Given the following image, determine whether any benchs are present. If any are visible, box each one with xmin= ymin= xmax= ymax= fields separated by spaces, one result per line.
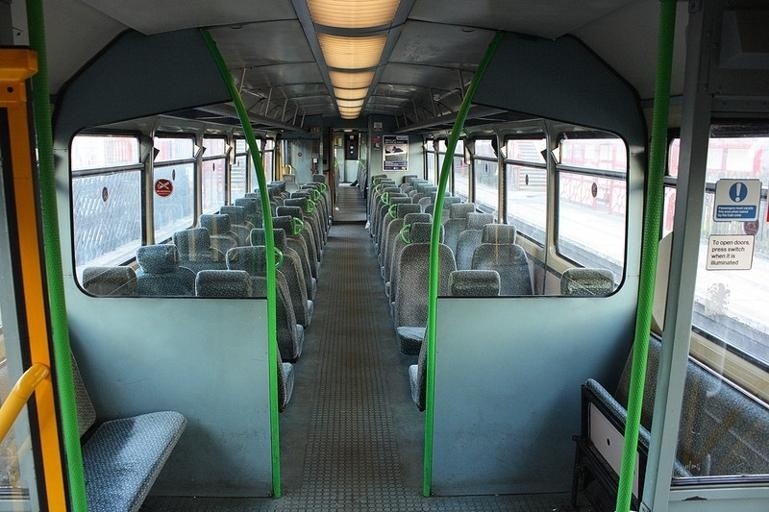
xmin=66 ymin=336 xmax=194 ymax=512
xmin=571 ymin=323 xmax=768 ymax=512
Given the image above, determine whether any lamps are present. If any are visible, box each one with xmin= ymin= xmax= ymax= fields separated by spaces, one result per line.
xmin=291 ymin=1 xmax=414 ymax=127
